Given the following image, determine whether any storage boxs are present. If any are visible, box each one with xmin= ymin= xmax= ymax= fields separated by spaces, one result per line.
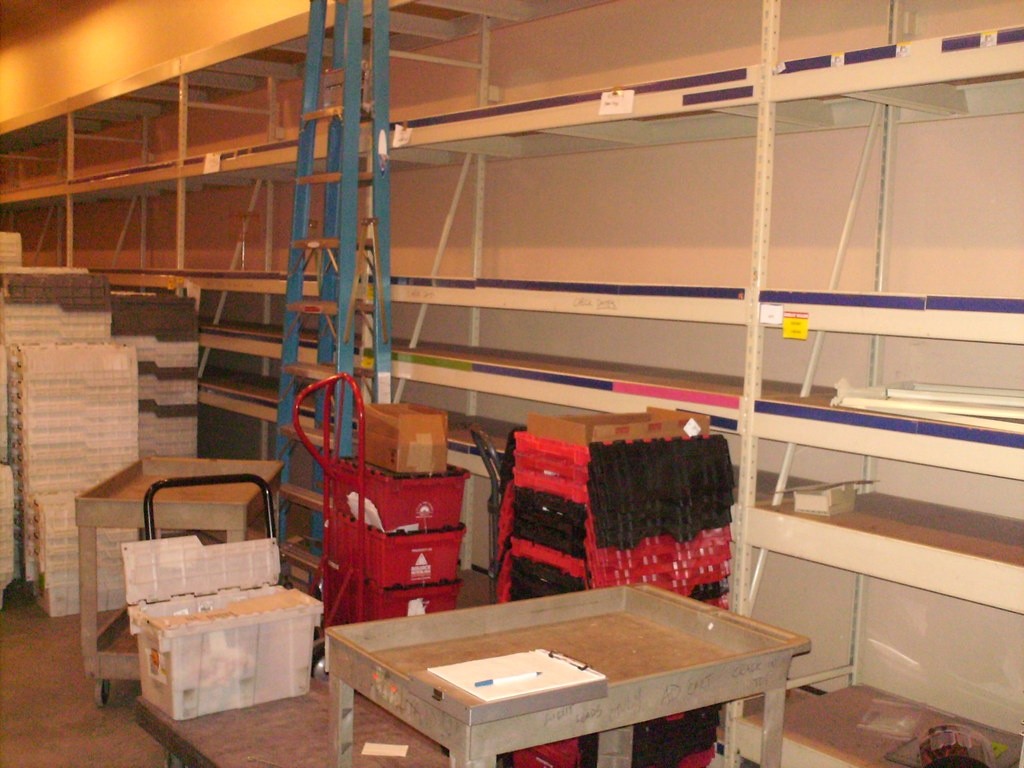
xmin=0 ymin=230 xmax=735 ymax=768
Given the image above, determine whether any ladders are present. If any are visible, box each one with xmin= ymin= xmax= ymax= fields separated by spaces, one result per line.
xmin=272 ymin=0 xmax=391 ymax=682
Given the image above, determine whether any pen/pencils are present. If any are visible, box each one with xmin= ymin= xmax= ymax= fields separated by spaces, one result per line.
xmin=475 ymin=671 xmax=542 ymax=687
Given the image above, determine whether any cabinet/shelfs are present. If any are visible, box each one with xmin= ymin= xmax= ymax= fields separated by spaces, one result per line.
xmin=1 ymin=0 xmax=1023 ymax=767
